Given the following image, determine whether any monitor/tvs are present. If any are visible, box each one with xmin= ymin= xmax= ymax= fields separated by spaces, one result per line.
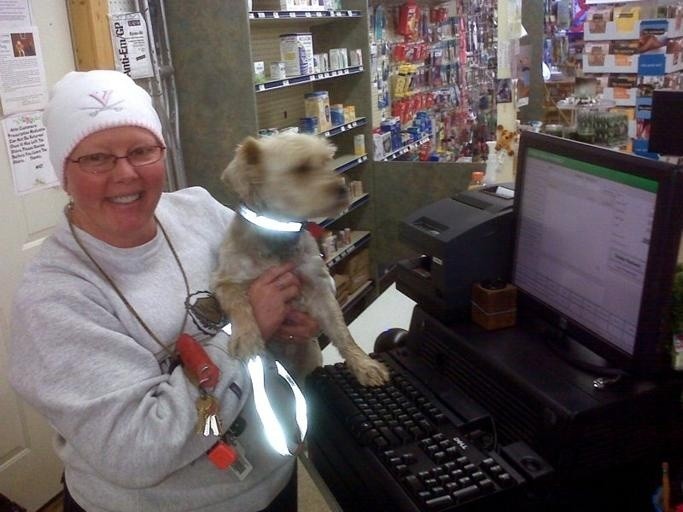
xmin=502 ymin=130 xmax=683 ymax=382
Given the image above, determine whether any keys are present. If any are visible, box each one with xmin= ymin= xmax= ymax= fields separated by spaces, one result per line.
xmin=194 ymin=393 xmax=224 ymax=436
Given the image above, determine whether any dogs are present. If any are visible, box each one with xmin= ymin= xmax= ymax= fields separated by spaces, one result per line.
xmin=209 ymin=127 xmax=390 ymax=458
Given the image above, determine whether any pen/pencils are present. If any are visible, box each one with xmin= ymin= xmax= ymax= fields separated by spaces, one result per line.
xmin=661 ymin=462 xmax=671 ymax=512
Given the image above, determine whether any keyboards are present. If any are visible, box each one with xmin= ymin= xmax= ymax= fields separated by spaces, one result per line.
xmin=309 ymin=351 xmax=525 ymax=512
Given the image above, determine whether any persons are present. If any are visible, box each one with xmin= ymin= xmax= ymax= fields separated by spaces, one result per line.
xmin=6 ymin=67 xmax=325 ymax=512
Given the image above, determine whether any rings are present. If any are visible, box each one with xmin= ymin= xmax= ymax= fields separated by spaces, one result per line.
xmin=288 ymin=334 xmax=296 ymax=345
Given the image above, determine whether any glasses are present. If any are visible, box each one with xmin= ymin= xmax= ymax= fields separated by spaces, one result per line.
xmin=67 ymin=145 xmax=166 ymax=175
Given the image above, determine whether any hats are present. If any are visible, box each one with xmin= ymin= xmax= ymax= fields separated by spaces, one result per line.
xmin=41 ymin=70 xmax=166 ymax=190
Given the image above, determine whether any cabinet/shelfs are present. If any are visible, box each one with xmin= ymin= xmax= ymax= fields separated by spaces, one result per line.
xmin=159 ymin=2 xmax=380 ymax=351
xmin=381 ymin=112 xmax=434 ymax=167
xmin=521 ymin=3 xmax=569 ymax=139
